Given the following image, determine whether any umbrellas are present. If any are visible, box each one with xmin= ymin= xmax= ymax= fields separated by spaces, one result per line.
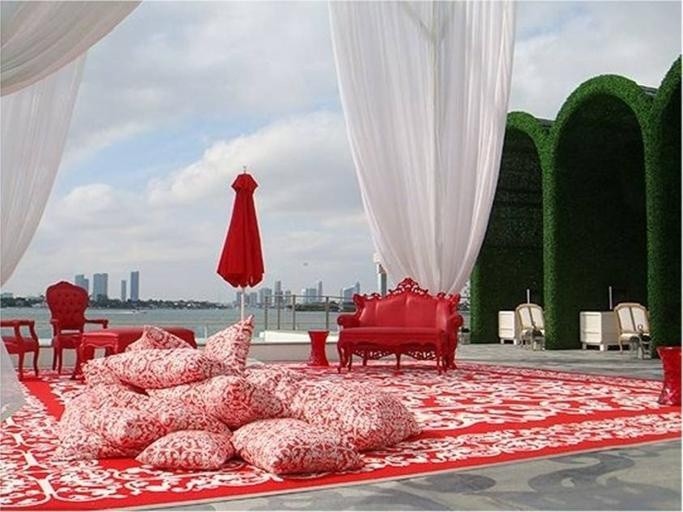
xmin=212 ymin=163 xmax=265 ymax=325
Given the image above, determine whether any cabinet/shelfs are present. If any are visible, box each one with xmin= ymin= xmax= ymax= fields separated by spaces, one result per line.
xmin=498 ymin=310 xmax=522 ymax=345
xmin=579 ymin=309 xmax=627 ymax=351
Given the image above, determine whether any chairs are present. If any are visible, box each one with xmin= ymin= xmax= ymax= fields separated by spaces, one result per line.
xmin=517 ymin=303 xmax=546 ymax=348
xmin=615 ymin=304 xmax=653 ymax=356
xmin=0 ymin=279 xmax=109 ymax=379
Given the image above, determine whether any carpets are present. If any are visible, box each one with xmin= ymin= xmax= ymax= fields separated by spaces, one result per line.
xmin=0 ymin=355 xmax=683 ymax=511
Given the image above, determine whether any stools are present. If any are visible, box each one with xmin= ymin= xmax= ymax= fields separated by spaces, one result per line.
xmin=657 ymin=346 xmax=682 ymax=406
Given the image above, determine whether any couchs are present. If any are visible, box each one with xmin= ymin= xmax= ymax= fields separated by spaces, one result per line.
xmin=334 ymin=276 xmax=463 ymax=376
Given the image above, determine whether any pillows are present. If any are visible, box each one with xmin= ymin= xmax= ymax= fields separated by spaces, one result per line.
xmin=52 ymin=313 xmax=295 ymax=458
xmin=136 ymin=430 xmax=232 ymax=472
xmin=294 ymin=382 xmax=421 ymax=446
xmin=232 ymin=421 xmax=363 ymax=472
